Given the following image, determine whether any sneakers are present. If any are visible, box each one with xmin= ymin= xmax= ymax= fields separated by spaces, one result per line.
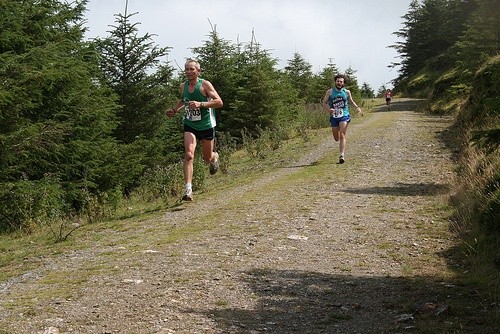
xmin=209 ymin=152 xmax=219 ymax=175
xmin=182 ymin=188 xmax=193 ymax=202
xmin=338 ymin=156 xmax=345 ymax=164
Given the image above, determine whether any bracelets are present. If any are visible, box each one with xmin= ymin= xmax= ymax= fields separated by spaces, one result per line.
xmin=172 ymin=107 xmax=177 ymax=114
xmin=355 ymin=106 xmax=358 ymax=109
xmin=200 ymin=102 xmax=204 ymax=107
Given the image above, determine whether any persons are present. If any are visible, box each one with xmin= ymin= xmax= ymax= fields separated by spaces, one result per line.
xmin=323 ymin=74 xmax=361 ymax=163
xmin=384 ymin=89 xmax=392 ymax=111
xmin=165 ymin=59 xmax=223 ymax=201
